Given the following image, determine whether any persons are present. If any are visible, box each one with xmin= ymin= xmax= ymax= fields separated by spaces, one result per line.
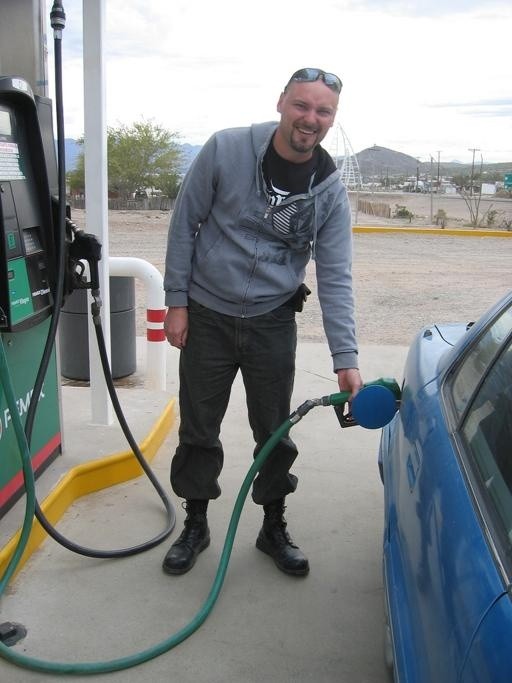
xmin=160 ymin=67 xmax=363 ymax=576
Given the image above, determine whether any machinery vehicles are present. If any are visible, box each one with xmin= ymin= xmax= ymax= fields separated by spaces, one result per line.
xmin=414 ymin=180 xmax=440 ymax=193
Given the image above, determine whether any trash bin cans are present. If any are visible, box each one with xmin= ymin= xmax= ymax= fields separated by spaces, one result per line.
xmin=58 ymin=276 xmax=136 ymax=381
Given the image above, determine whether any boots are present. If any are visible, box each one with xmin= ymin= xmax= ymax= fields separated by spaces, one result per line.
xmin=163 ymin=499 xmax=210 ymax=575
xmin=256 ymin=497 xmax=309 ymax=575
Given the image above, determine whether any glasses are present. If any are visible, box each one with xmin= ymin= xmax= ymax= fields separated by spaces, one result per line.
xmin=283 ymin=67 xmax=343 ymax=95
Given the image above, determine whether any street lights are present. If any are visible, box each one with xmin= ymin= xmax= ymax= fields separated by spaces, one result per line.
xmin=468 ymin=149 xmax=480 ymax=196
xmin=416 ymin=156 xmax=421 ymax=193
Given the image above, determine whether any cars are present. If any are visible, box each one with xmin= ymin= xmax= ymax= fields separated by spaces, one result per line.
xmin=374 ymin=288 xmax=512 ymax=683
